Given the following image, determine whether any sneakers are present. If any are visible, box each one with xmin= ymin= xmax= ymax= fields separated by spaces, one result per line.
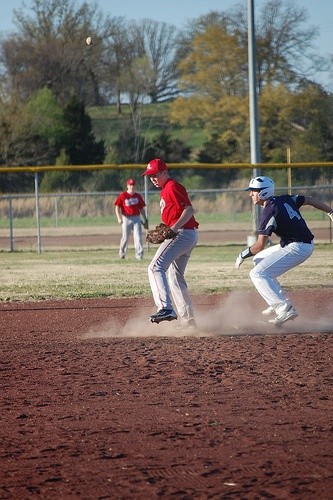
xmin=268 ymin=305 xmax=298 ymax=327
xmin=150 ymin=309 xmax=178 ymax=324
xmin=262 ymin=307 xmax=275 ymax=315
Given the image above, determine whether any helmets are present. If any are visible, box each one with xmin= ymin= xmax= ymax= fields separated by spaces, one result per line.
xmin=245 ymin=176 xmax=275 ymax=200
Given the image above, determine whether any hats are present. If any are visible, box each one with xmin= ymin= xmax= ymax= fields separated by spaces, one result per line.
xmin=141 ymin=159 xmax=168 ymax=176
xmin=127 ymin=179 xmax=136 ymax=185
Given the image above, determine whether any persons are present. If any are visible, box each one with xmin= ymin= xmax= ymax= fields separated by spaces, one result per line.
xmin=234 ymin=177 xmax=333 ymax=324
xmin=114 ymin=180 xmax=148 ymax=262
xmin=141 ymin=159 xmax=199 ymax=333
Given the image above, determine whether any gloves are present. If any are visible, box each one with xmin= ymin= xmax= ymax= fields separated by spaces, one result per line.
xmin=235 ymin=247 xmax=256 ymax=270
xmin=328 ymin=213 xmax=333 ymax=221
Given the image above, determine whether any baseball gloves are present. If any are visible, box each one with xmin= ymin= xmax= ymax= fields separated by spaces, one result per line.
xmin=145 ymin=223 xmax=178 ymax=244
